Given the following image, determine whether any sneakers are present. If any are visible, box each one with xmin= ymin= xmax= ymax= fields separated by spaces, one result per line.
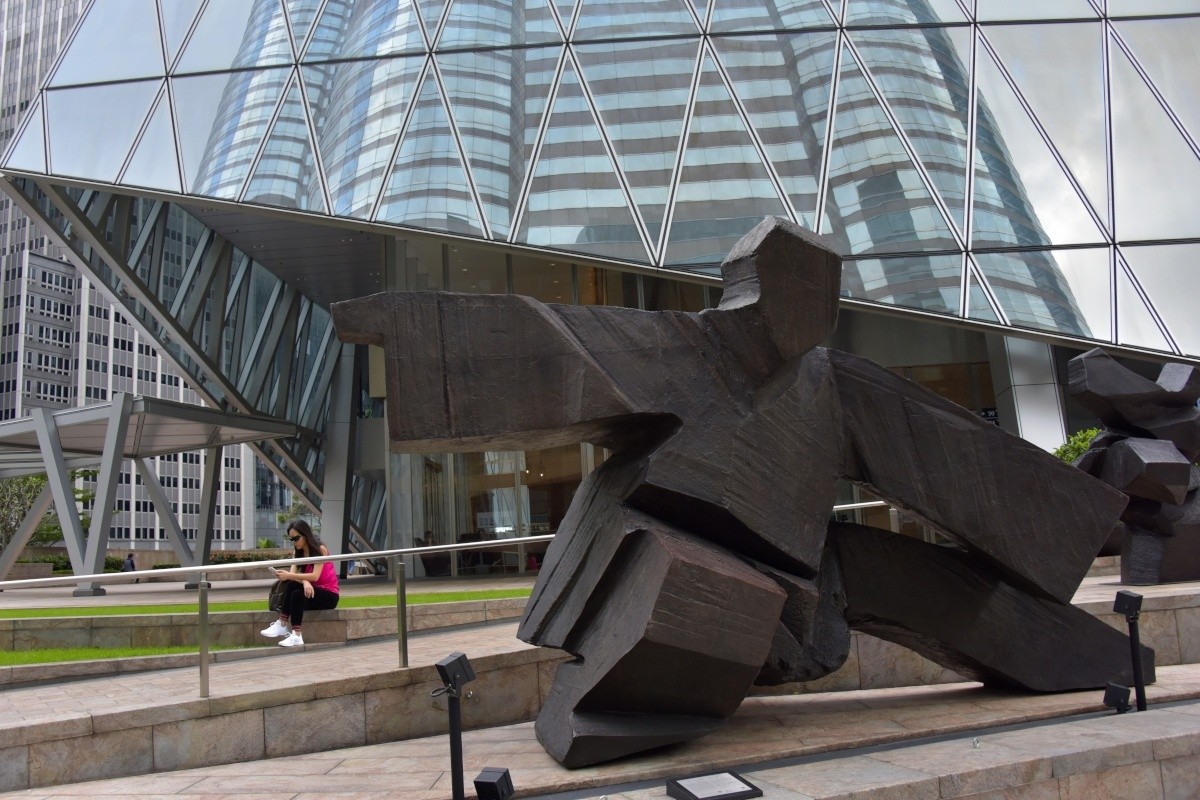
xmin=278 ymin=629 xmax=305 ymax=647
xmin=260 ymin=619 xmax=289 ymax=638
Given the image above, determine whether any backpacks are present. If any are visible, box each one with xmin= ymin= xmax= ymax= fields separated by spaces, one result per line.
xmin=268 ymin=580 xmax=290 ymax=612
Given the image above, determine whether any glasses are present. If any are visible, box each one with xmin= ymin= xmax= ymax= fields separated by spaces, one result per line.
xmin=288 ymin=534 xmax=304 ymax=542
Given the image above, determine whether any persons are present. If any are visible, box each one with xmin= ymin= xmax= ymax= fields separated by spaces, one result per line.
xmin=124 ymin=553 xmax=140 ymax=584
xmin=423 ymin=531 xmax=450 ymax=558
xmin=260 ymin=520 xmax=339 ymax=647
xmin=347 ymin=560 xmax=355 ymax=576
xmin=210 ymin=553 xmax=216 ymax=561
xmin=457 ymin=534 xmax=474 ymax=566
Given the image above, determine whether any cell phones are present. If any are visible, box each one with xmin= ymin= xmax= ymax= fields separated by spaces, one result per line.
xmin=268 ymin=567 xmax=277 ymax=574
